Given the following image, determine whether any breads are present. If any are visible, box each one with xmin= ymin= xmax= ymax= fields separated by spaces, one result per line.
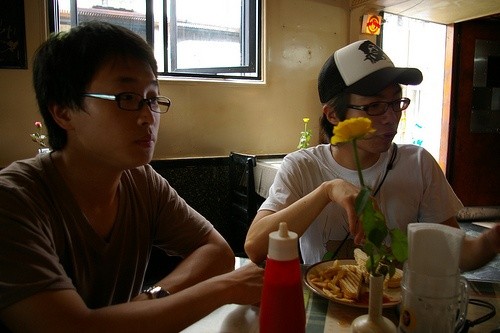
xmin=339 ymin=248 xmax=404 ymax=299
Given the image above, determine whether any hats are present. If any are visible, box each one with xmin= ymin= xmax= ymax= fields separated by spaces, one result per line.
xmin=318 ymin=40 xmax=423 ymax=102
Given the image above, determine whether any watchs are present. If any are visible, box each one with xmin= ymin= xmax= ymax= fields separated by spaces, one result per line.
xmin=143 ymin=286 xmax=170 ymax=298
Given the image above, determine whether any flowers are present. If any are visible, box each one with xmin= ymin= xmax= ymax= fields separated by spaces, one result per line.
xmin=31 ymin=122 xmax=46 ymax=150
xmin=331 ymin=117 xmax=409 ymax=278
xmin=298 ymin=117 xmax=312 ymax=149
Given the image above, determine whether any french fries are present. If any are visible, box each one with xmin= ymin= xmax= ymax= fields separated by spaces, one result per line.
xmin=309 ymin=259 xmax=357 ymax=303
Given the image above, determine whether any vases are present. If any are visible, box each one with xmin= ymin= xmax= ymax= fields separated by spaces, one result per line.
xmin=350 ymin=273 xmax=397 ymax=333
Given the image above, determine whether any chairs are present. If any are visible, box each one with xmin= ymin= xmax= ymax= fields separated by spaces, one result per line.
xmin=227 ymin=152 xmax=256 ymax=252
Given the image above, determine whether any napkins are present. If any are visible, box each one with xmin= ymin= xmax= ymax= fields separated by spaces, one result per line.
xmin=407 ymin=222 xmax=465 ymax=297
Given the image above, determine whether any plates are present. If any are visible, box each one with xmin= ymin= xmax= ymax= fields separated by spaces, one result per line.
xmin=303 ymin=259 xmax=405 ymax=308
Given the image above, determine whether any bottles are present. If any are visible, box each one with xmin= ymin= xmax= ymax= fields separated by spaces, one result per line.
xmin=258 ymin=222 xmax=306 ymax=333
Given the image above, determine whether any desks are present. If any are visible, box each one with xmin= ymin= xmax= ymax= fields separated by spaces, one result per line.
xmin=253 ymin=158 xmax=286 ymax=198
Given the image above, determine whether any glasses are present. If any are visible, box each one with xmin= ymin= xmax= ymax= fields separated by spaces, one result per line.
xmin=80 ymin=92 xmax=171 ymax=115
xmin=345 ymin=96 xmax=410 ymax=116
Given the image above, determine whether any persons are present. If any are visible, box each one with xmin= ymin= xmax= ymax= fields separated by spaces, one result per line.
xmin=0 ymin=22 xmax=264 ymax=332
xmin=243 ymin=40 xmax=464 ymax=263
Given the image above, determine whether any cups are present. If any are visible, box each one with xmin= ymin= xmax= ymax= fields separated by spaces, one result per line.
xmin=397 ymin=274 xmax=469 ymax=333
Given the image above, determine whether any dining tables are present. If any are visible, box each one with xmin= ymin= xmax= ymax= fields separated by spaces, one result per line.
xmin=181 ymin=257 xmax=500 ymax=333
